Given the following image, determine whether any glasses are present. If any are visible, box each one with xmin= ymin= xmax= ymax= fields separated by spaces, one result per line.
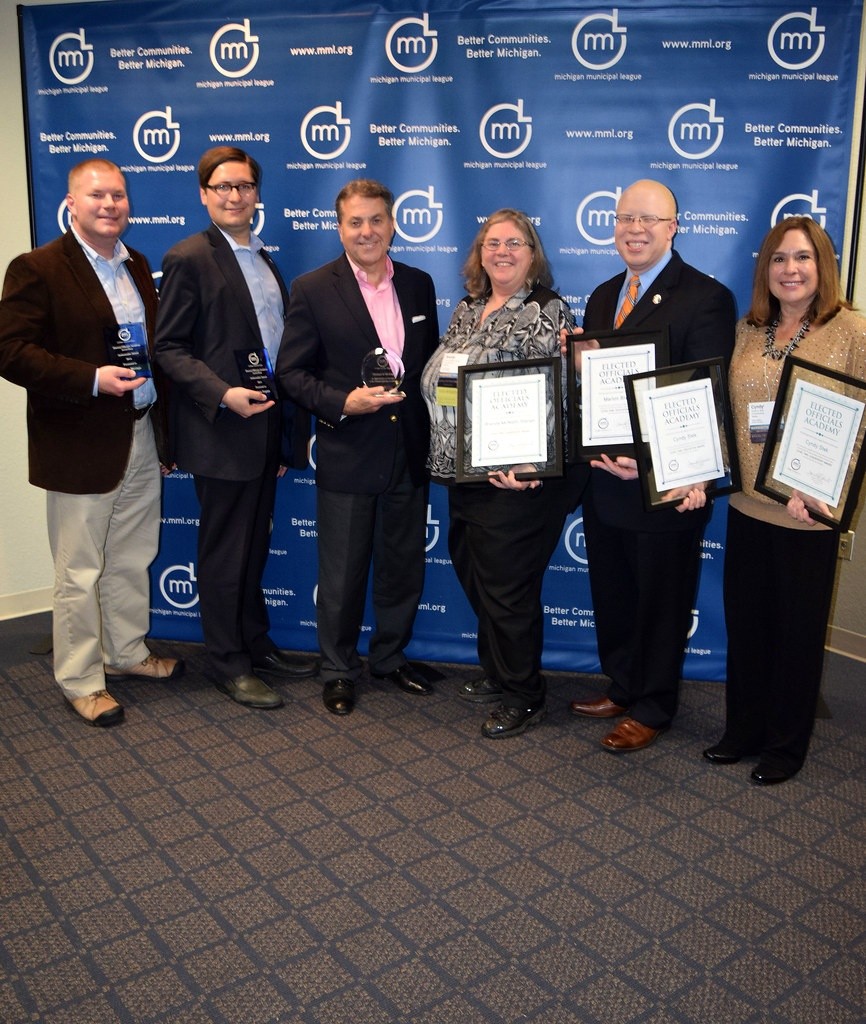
xmin=206 ymin=182 xmax=257 ymax=197
xmin=614 ymin=214 xmax=671 ymax=229
xmin=481 ymin=238 xmax=535 ymax=251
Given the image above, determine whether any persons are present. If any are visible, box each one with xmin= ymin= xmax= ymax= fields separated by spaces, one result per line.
xmin=1 ymin=156 xmax=190 ymax=726
xmin=276 ymin=179 xmax=441 ymax=716
xmin=153 ymin=144 xmax=321 ymax=710
xmin=559 ymin=179 xmax=740 ymax=755
xmin=676 ymin=217 xmax=865 ymax=786
xmin=423 ymin=207 xmax=582 ymax=741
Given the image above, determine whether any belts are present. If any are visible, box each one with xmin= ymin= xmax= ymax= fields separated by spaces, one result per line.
xmin=134 ymin=404 xmax=151 ymax=420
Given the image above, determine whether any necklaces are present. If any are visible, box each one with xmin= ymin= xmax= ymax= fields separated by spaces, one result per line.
xmin=762 ymin=312 xmax=810 ymax=362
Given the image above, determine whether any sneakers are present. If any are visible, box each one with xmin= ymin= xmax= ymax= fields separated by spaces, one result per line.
xmin=481 ymin=704 xmax=547 ymax=739
xmin=63 ymin=690 xmax=125 ymax=727
xmin=458 ymin=675 xmax=505 ymax=702
xmin=104 ymin=653 xmax=185 ymax=683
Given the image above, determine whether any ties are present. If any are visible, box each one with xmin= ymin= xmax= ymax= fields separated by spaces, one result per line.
xmin=613 ymin=275 xmax=641 ymax=329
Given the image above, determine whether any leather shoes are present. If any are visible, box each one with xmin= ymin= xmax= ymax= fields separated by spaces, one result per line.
xmin=323 ymin=678 xmax=354 ymax=713
xmin=371 ymin=663 xmax=433 ymax=695
xmin=251 ymin=650 xmax=319 ymax=678
xmin=751 ymin=763 xmax=802 ymax=786
xmin=703 ymin=745 xmax=758 ymax=764
xmin=570 ymin=694 xmax=631 ymax=718
xmin=215 ymin=673 xmax=282 ymax=708
xmin=600 ymin=715 xmax=672 ymax=752
